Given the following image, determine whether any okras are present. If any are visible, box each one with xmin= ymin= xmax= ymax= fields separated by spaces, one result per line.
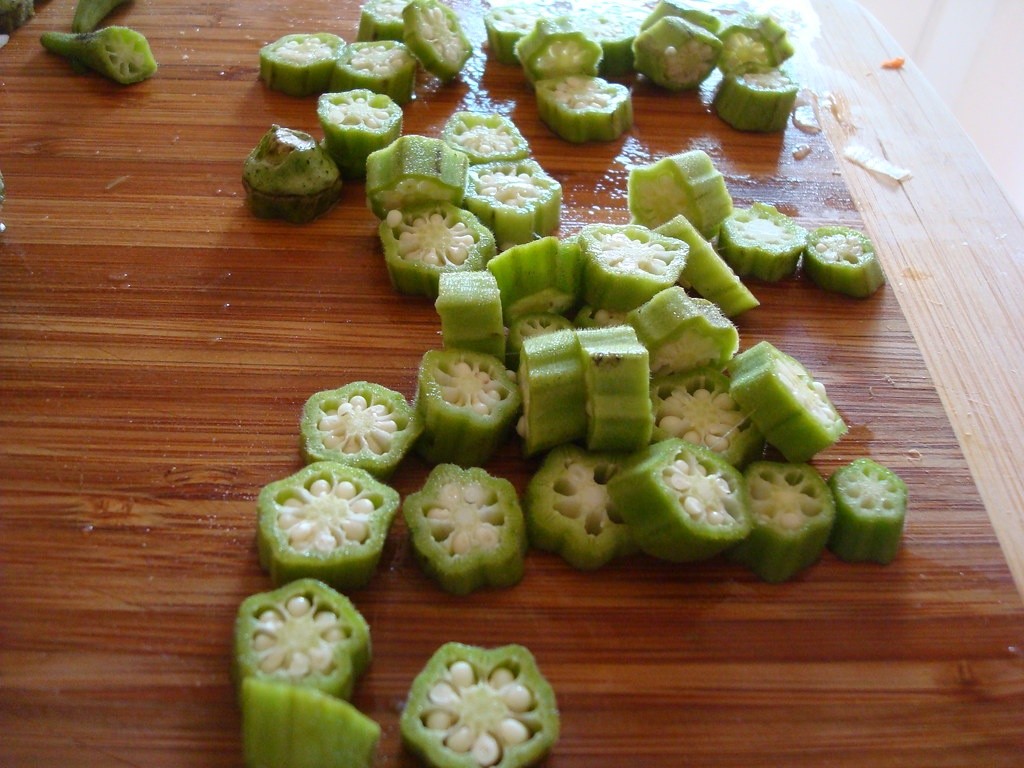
xmin=39 ymin=0 xmax=906 ymax=768
